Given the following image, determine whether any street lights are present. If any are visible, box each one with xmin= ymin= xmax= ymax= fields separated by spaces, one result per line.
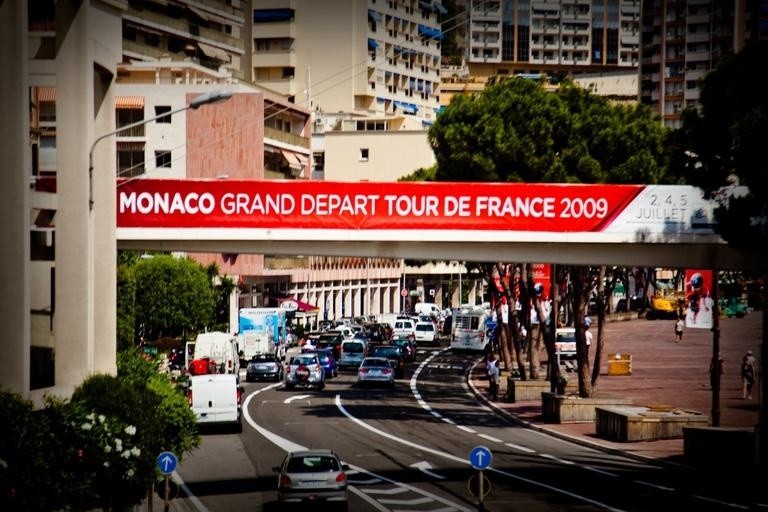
xmin=86 ymin=84 xmax=235 ymax=381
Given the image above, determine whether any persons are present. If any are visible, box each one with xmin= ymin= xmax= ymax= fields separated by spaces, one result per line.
xmin=739 ymin=355 xmax=756 ymax=401
xmin=708 ymin=350 xmax=726 ymax=391
xmin=685 ymin=272 xmax=711 ymax=326
xmin=487 ymin=359 xmax=501 ymax=402
xmin=486 ymin=352 xmax=497 ymax=384
xmin=746 ymin=350 xmax=756 ymax=378
xmin=215 ymin=362 xmax=226 ymax=375
xmin=583 ymin=324 xmax=593 ymax=360
xmin=430 ymin=302 xmax=453 ymax=334
xmin=583 ymin=314 xmax=593 ymax=331
xmin=529 ymin=282 xmax=549 ymax=323
xmin=673 ymin=316 xmax=685 ymax=344
xmin=517 ymin=325 xmax=529 ymax=352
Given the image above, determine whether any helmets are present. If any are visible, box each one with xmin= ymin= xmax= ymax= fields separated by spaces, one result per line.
xmin=690 ymin=273 xmax=703 ymax=288
xmin=534 ymin=283 xmax=543 ymax=294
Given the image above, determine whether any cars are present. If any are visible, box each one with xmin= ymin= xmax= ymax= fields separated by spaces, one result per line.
xmin=615 ymin=295 xmax=650 ymax=312
xmin=271 ymin=448 xmax=352 ymax=512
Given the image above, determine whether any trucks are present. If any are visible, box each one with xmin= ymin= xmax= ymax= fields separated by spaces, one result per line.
xmin=183 ymin=337 xmax=245 ymax=433
xmin=237 ymin=307 xmax=288 ymax=362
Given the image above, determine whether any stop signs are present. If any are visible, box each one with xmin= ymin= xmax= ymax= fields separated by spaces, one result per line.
xmin=400 ymin=288 xmax=408 ymax=297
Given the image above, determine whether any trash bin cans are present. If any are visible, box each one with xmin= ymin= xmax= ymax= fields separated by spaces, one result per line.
xmin=607 ymin=353 xmax=632 ymax=375
